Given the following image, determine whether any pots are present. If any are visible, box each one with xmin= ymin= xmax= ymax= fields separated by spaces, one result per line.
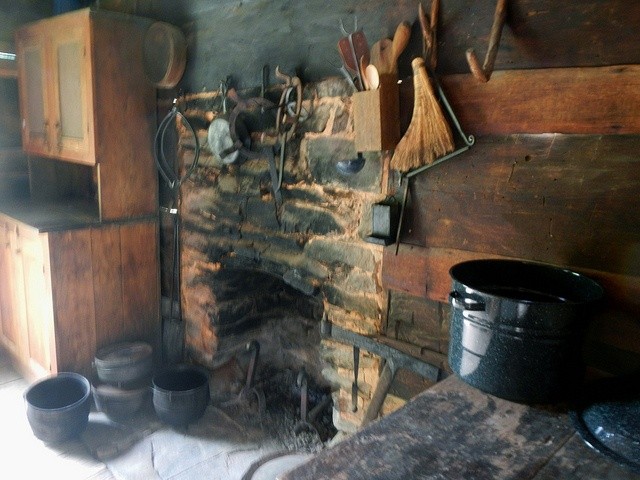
xmin=149 ymin=368 xmax=209 ymax=427
xmin=446 ymin=258 xmax=607 ymax=406
xmin=23 ymin=372 xmax=92 ymax=444
xmin=206 ymin=81 xmax=249 ymax=166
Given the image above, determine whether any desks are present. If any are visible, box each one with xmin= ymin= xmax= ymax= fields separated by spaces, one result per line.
xmin=279 ymin=374 xmax=639 ymax=480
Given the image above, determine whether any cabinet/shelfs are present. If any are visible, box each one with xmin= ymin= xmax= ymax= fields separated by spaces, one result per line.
xmin=15 ymin=10 xmax=158 ymax=166
xmin=1 ymin=214 xmax=162 ymax=384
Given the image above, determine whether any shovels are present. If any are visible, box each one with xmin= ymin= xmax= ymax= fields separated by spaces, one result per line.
xmin=339 ymin=31 xmax=370 ymax=72
xmin=372 ymin=39 xmax=396 ymax=75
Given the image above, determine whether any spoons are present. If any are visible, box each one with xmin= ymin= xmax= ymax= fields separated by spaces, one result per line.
xmin=365 ymin=64 xmax=380 ymax=90
xmin=335 ymin=94 xmax=365 ymax=175
